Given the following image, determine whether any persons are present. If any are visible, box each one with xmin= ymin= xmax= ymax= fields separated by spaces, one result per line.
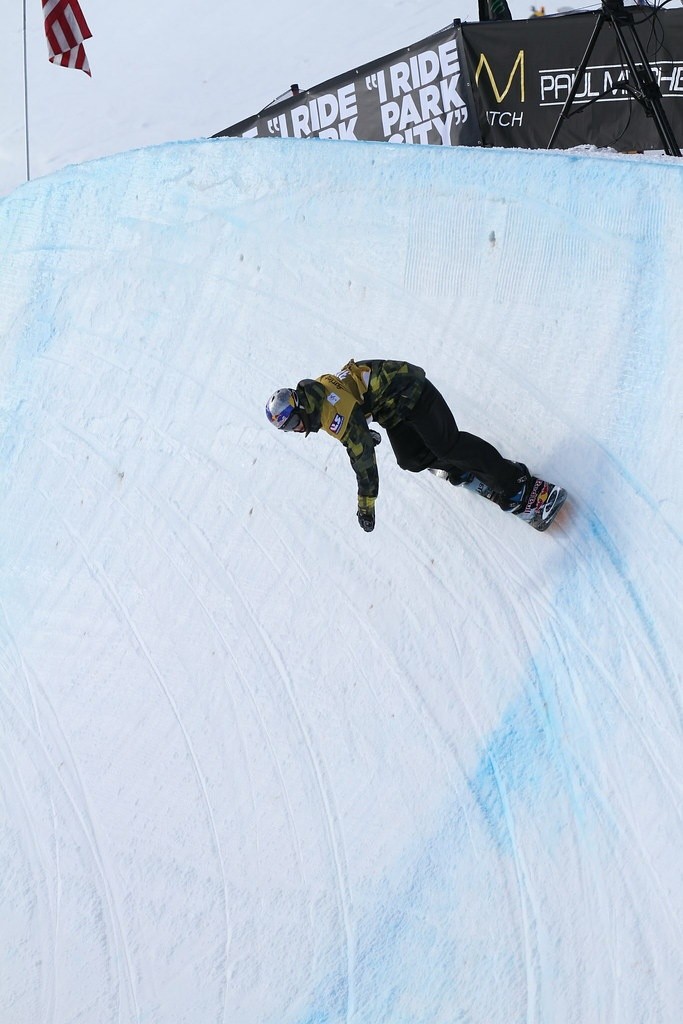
xmin=265 ymin=359 xmax=528 ymax=532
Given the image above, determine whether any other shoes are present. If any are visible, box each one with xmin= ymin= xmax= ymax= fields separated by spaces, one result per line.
xmin=499 ymin=462 xmax=531 ymax=512
xmin=446 ymin=468 xmax=475 ymax=485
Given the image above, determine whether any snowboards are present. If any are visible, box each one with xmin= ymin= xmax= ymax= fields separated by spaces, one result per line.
xmin=428 ymin=455 xmax=569 ymax=533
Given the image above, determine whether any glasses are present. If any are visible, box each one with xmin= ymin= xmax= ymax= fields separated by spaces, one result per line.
xmin=283 ymin=413 xmax=301 ymax=432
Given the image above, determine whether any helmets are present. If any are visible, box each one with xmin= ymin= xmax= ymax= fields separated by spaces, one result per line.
xmin=266 ymin=388 xmax=300 ymax=429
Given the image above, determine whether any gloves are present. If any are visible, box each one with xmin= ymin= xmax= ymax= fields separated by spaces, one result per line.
xmin=356 ymin=494 xmax=379 ymax=532
xmin=369 ymin=430 xmax=381 ymax=446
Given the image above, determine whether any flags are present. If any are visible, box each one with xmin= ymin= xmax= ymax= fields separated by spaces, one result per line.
xmin=41 ymin=0 xmax=92 ymax=77
